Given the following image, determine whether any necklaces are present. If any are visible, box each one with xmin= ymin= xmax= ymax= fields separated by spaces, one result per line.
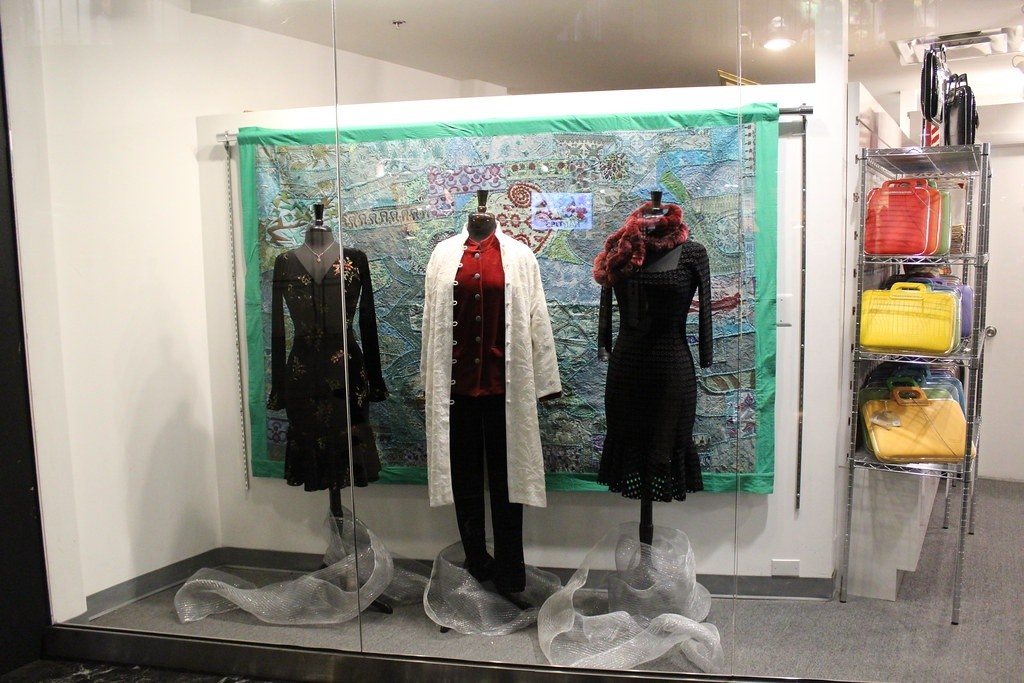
xmin=303 ymin=238 xmax=335 ymax=263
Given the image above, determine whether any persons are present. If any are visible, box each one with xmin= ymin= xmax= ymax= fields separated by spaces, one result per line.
xmin=267 ymin=224 xmax=389 ymax=492
xmin=419 ymin=213 xmax=562 ymax=593
xmin=598 ymin=207 xmax=713 ymax=501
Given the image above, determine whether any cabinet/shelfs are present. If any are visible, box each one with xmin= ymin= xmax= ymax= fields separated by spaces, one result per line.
xmin=838 ymin=142 xmax=992 ymax=628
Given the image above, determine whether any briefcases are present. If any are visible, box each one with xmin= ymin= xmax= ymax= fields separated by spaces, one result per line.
xmin=863 ymin=361 xmax=978 ymax=463
xmin=921 ymin=45 xmax=979 ymax=144
xmin=862 ymin=273 xmax=973 ymax=353
xmin=868 ymin=175 xmax=955 ymax=255
